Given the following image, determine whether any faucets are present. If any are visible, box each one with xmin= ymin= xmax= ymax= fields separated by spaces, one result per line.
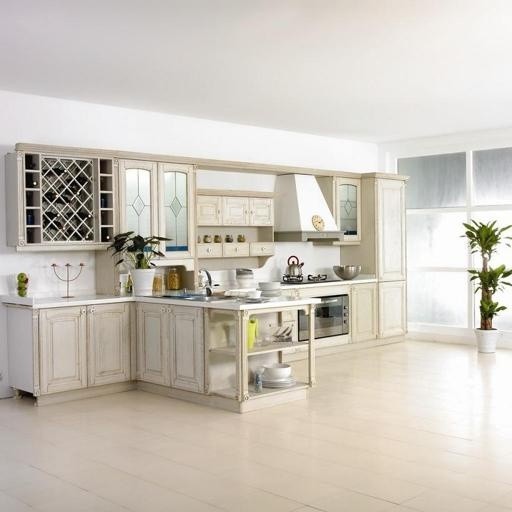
xmin=199 ymin=269 xmax=211 ymax=287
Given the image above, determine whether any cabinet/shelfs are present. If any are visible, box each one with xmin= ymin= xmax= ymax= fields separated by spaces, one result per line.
xmin=118 ymin=151 xmax=195 ymax=272
xmin=135 ymin=298 xmax=205 ymax=405
xmin=4 ymin=142 xmax=118 ymax=253
xmin=313 ymin=172 xmax=410 ymax=282
xmin=350 ymin=283 xmax=408 ymax=349
xmin=1 ymin=299 xmax=134 ymax=407
xmin=205 ymin=298 xmax=321 ymax=414
xmin=196 ymin=189 xmax=275 ymax=258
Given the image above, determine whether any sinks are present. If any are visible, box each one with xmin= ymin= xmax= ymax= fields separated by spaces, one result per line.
xmin=184 ymin=297 xmax=224 ymax=302
xmin=163 ymin=295 xmax=203 ymax=298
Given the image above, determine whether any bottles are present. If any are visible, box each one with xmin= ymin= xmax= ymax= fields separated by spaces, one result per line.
xmin=198 ymin=234 xmax=246 ymax=244
xmin=248 ymin=321 xmax=256 ymax=350
xmin=166 ymin=268 xmax=182 ymax=290
xmin=254 ymin=365 xmax=263 ymax=394
xmin=25 ymin=161 xmax=111 ymax=242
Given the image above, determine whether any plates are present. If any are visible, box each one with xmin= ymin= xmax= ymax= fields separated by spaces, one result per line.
xmin=245 ymin=296 xmax=263 ymax=302
xmin=261 ymin=377 xmax=296 ymax=389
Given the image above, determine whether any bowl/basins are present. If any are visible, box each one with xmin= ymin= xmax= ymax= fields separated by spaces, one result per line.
xmin=235 ymin=268 xmax=254 ymax=287
xmin=333 ymin=264 xmax=362 ymax=280
xmin=248 ymin=290 xmax=262 ymax=298
xmin=258 ymin=281 xmax=280 ymax=290
xmin=261 ymin=362 xmax=292 ymax=381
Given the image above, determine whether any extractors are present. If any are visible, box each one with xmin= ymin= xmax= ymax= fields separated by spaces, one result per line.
xmin=272 ymin=173 xmax=348 ymax=243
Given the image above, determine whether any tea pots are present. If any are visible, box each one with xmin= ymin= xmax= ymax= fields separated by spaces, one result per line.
xmin=285 ymin=255 xmax=304 ymax=276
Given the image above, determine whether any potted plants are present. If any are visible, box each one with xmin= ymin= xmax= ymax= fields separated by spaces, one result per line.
xmin=459 ymin=220 xmax=512 ymax=353
xmin=108 ymin=232 xmax=174 ymax=296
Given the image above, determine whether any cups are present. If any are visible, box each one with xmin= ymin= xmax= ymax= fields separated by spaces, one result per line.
xmin=151 ymin=271 xmax=165 ymax=298
xmin=118 ymin=273 xmax=130 ymax=295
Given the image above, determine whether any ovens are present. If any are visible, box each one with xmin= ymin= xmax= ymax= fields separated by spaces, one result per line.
xmin=297 ymin=293 xmax=351 ymax=343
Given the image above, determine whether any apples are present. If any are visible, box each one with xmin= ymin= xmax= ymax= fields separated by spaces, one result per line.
xmin=18 ymin=273 xmax=27 ymax=296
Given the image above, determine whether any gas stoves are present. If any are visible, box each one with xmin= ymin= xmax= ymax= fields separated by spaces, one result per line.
xmin=280 ymin=273 xmax=341 ymax=284
